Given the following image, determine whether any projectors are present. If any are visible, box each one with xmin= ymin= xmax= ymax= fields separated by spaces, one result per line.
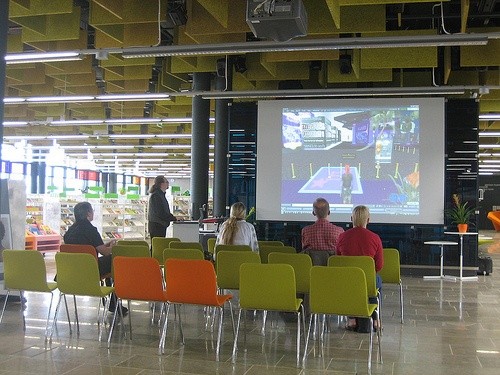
xmin=245 ymin=1 xmax=308 ymax=43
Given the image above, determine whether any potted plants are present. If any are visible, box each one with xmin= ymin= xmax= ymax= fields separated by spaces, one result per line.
xmin=443 ymin=193 xmax=478 ymax=232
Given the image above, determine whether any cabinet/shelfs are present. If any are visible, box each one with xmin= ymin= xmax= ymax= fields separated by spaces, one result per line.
xmin=27 ymin=191 xmax=190 ymax=239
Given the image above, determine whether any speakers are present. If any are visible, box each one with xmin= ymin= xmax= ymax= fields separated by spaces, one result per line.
xmin=233 ymin=56 xmax=247 ymax=73
xmin=339 ymin=55 xmax=352 ymax=74
xmin=216 ymin=58 xmax=225 ymax=78
xmin=168 ymin=3 xmax=188 ymax=26
xmin=312 ymin=60 xmax=322 ymax=70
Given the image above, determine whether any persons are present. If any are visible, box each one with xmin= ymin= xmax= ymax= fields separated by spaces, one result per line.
xmin=334 ymin=205 xmax=384 ymax=333
xmin=302 ymin=198 xmax=344 ymax=256
xmin=0 ymin=221 xmax=7 ymax=262
xmin=341 ymin=163 xmax=353 ymax=204
xmin=148 ymin=175 xmax=186 ymax=259
xmin=213 ymin=202 xmax=261 ymax=264
xmin=54 ymin=202 xmax=128 ymax=315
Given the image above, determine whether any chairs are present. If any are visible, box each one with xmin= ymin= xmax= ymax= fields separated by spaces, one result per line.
xmin=49 ymin=237 xmax=404 ymax=375
xmin=0 ymin=249 xmax=57 ymax=345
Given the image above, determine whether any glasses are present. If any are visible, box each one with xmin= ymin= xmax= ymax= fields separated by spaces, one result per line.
xmin=163 ymin=180 xmax=168 ymax=182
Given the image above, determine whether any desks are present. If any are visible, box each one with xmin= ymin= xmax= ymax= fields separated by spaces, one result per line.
xmin=444 ymin=232 xmax=479 ymax=282
xmin=423 ymin=241 xmax=459 ymax=281
xmin=170 ymin=221 xmax=200 ymax=242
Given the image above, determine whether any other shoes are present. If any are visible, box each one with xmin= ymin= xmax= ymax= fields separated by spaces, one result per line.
xmin=347 ymin=324 xmax=356 ymax=331
xmin=375 ymin=326 xmax=383 ymax=331
xmin=109 ymin=301 xmax=128 ymax=313
